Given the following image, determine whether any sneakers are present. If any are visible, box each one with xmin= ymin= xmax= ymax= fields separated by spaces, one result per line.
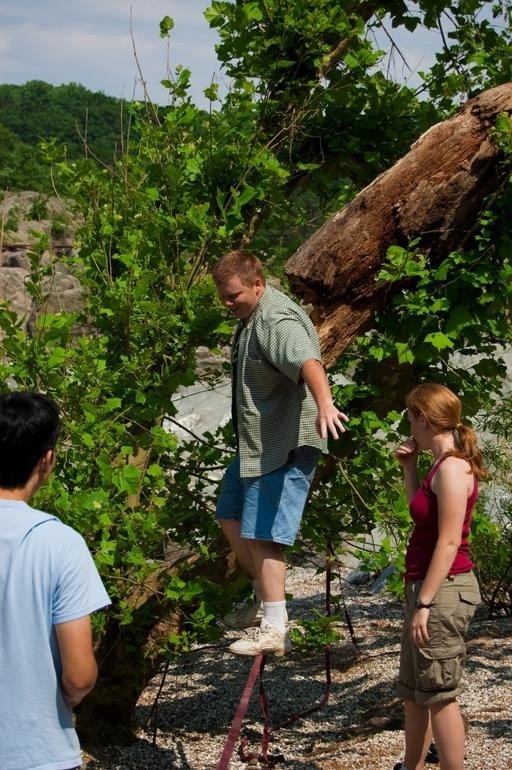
xmin=228 ymin=626 xmax=291 ymax=656
xmin=223 ymin=603 xmax=262 ymax=628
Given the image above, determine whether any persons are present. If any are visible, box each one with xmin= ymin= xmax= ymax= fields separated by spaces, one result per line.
xmin=393 ymin=383 xmax=483 ymax=770
xmin=0 ymin=390 xmax=112 ymax=770
xmin=214 ymin=250 xmax=349 ymax=657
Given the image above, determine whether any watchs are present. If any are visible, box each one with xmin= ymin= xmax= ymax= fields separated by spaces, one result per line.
xmin=416 ymin=600 xmax=430 ymax=608
